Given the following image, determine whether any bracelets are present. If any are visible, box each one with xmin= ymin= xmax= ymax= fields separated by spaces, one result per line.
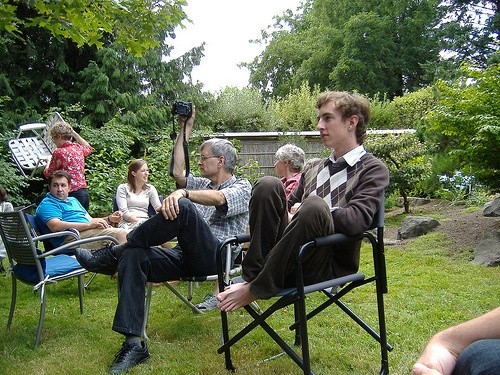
xmin=183 ymin=188 xmax=189 ymax=198
xmin=108 ymin=216 xmax=113 ymax=223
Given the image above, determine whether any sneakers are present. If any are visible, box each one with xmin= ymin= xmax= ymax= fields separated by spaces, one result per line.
xmin=76 ymin=241 xmax=119 ymax=275
xmin=193 ymin=292 xmax=221 ymax=313
xmin=108 ymin=341 xmax=151 ymax=375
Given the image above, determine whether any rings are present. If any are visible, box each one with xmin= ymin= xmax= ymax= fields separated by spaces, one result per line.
xmin=170 ymin=204 xmax=174 ymax=206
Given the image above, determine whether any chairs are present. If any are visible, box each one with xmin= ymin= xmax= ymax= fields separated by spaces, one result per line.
xmin=0 ymin=212 xmax=91 ymax=351
xmin=114 ymin=196 xmax=163 ymax=229
xmin=26 ymin=213 xmax=99 ymax=289
xmin=13 ymin=202 xmax=36 ymax=239
xmin=8 ymin=112 xmax=73 ymax=181
xmin=141 ymin=223 xmax=265 ymax=341
xmin=216 ymin=189 xmax=394 ymax=375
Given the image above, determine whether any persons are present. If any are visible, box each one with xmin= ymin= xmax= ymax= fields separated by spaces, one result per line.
xmin=35 ymin=170 xmax=179 ymax=286
xmin=74 ymin=105 xmax=253 ymax=374
xmin=43 ymin=122 xmax=92 ymax=212
xmin=216 ymin=91 xmax=389 ymax=312
xmin=0 ymin=186 xmax=13 ymax=271
xmin=410 ymin=307 xmax=500 ymax=375
xmin=194 ymin=144 xmax=306 ymax=312
xmin=116 ymin=159 xmax=172 ymax=249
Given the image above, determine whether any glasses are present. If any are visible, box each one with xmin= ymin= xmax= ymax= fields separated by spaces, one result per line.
xmin=197 ymin=154 xmax=220 ymax=161
xmin=51 ymin=136 xmax=57 ymax=140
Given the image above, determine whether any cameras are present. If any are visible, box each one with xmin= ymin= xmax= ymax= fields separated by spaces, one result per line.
xmin=173 ymin=101 xmax=192 ymax=117
